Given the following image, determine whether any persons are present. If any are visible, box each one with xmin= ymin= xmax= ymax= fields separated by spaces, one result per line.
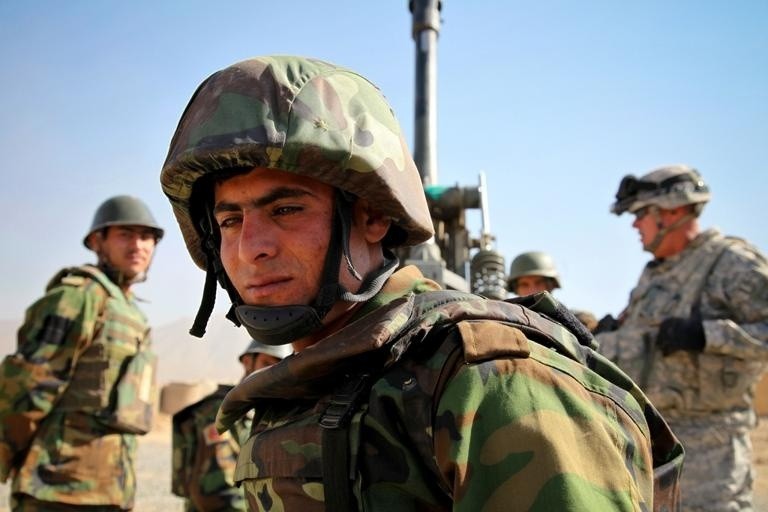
xmin=601 ymin=163 xmax=766 ymax=512
xmin=505 ymin=249 xmax=595 ymax=333
xmin=0 ymin=194 xmax=164 ymax=512
xmin=163 ymin=337 xmax=295 ymax=510
xmin=161 ymin=54 xmax=686 ymax=511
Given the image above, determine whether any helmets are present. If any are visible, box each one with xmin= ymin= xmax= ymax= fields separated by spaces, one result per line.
xmin=158 ymin=52 xmax=438 ymax=274
xmin=626 ymin=160 xmax=714 ymax=217
xmin=501 ymin=250 xmax=562 ymax=294
xmin=81 ymin=196 xmax=164 ymax=250
xmin=235 ymin=338 xmax=294 ymax=363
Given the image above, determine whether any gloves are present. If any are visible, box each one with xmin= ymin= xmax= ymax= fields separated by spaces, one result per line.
xmin=651 ymin=313 xmax=708 ymax=360
